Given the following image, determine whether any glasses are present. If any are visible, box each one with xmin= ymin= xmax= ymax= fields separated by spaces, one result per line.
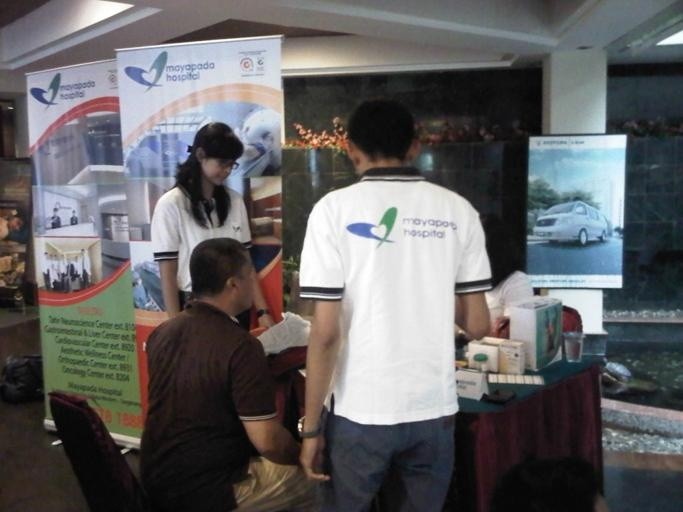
xmin=219 ymin=159 xmax=238 ymax=169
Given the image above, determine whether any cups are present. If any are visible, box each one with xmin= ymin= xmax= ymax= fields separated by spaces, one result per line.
xmin=565 ymin=332 xmax=586 ymax=362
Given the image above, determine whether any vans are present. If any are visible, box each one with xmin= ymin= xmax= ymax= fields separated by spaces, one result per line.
xmin=532 ymin=201 xmax=607 ymax=245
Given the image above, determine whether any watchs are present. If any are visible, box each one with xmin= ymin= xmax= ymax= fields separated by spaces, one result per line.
xmin=254 ymin=309 xmax=271 ymax=318
xmin=297 ymin=415 xmax=322 ymax=438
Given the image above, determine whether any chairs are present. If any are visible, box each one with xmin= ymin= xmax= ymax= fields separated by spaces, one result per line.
xmin=47 ymin=389 xmax=156 ymax=512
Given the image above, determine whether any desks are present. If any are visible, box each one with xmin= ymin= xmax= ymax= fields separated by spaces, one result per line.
xmin=270 ymin=353 xmax=607 ymax=512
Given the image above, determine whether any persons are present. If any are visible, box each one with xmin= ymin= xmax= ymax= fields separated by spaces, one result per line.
xmin=149 ymin=122 xmax=276 ymax=457
xmin=139 ymin=236 xmax=331 ymax=512
xmin=50 ymin=206 xmax=62 ymax=228
xmin=297 ymin=99 xmax=494 ymax=512
xmin=70 ymin=209 xmax=78 ymax=225
xmin=41 ymin=263 xmax=91 ymax=294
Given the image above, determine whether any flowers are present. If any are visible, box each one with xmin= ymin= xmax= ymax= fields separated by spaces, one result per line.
xmin=279 ymin=116 xmax=523 ymax=150
